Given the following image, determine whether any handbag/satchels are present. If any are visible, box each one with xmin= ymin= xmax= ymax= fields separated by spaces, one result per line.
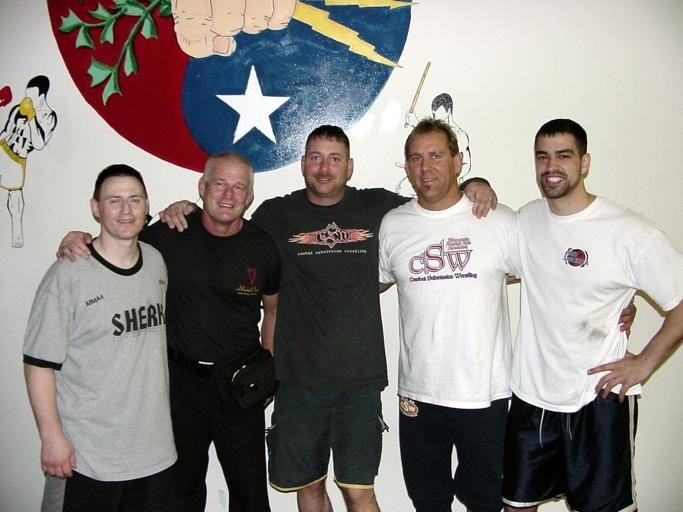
xmin=210 ymin=349 xmax=280 ymax=409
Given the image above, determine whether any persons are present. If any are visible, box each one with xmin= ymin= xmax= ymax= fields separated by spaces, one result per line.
xmin=504 ymin=118 xmax=683 ymax=512
xmin=55 ymin=153 xmax=284 ymax=511
xmin=156 ymin=123 xmax=498 ymax=511
xmin=169 ymin=1 xmax=300 ymax=60
xmin=377 ymin=113 xmax=636 ymax=512
xmin=0 ymin=75 xmax=58 ymax=249
xmin=21 ymin=164 xmax=180 ymax=510
xmin=394 ymin=92 xmax=472 ymax=196
